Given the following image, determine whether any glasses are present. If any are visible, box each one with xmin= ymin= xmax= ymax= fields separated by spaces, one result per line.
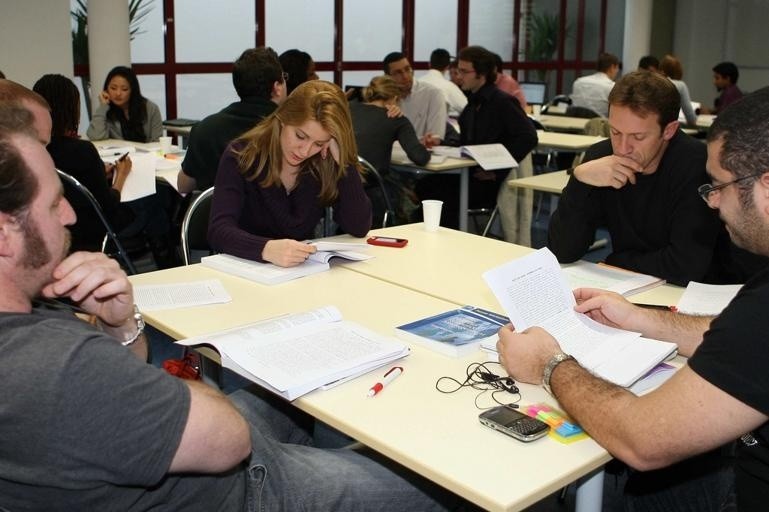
xmin=387 ymin=66 xmax=414 ymax=77
xmin=696 ymin=171 xmax=758 ymax=206
xmin=457 ymin=67 xmax=480 ymax=75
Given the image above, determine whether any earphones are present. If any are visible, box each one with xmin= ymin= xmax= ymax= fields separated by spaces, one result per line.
xmin=510 ymin=386 xmax=519 ymax=394
xmin=506 ymin=378 xmax=515 ymax=385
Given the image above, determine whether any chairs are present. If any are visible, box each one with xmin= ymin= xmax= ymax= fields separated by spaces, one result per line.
xmin=52 ymin=94 xmax=769 ymax=512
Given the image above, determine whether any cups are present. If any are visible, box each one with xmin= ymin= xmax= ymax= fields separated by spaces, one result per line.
xmin=420 ymin=199 xmax=444 ymax=230
xmin=159 ymin=137 xmax=172 ymax=154
xmin=532 ymin=104 xmax=541 ymax=117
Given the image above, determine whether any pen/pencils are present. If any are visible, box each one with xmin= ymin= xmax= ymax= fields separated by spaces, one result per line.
xmin=113 ymin=152 xmax=130 ymax=168
xmin=367 ymin=365 xmax=404 ymax=397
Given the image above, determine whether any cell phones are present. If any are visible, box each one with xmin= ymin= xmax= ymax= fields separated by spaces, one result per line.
xmin=478 ymin=405 xmax=551 ymax=443
xmin=367 ymin=236 xmax=408 ymax=248
